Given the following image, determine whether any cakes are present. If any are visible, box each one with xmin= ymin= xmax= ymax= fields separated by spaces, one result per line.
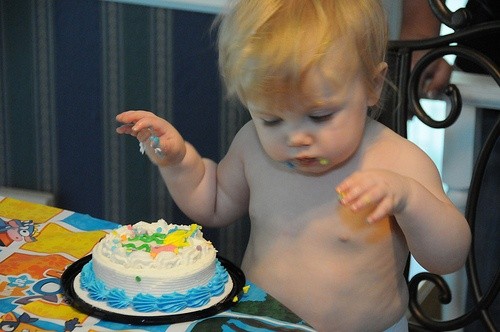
xmin=79 ymin=218 xmax=230 ymax=314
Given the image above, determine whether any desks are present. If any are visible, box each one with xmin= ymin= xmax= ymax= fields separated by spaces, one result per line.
xmin=0 ymin=191 xmax=319 ymax=332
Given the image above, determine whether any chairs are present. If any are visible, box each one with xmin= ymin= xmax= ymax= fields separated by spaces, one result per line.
xmin=367 ymin=0 xmax=500 ymax=332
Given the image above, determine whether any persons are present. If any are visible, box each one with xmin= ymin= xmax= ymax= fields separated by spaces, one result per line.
xmin=381 ymin=0 xmax=452 ymax=98
xmin=114 ymin=0 xmax=472 ymax=332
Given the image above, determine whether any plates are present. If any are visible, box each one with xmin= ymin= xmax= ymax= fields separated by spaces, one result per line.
xmin=60 ymin=252 xmax=246 ymax=326
